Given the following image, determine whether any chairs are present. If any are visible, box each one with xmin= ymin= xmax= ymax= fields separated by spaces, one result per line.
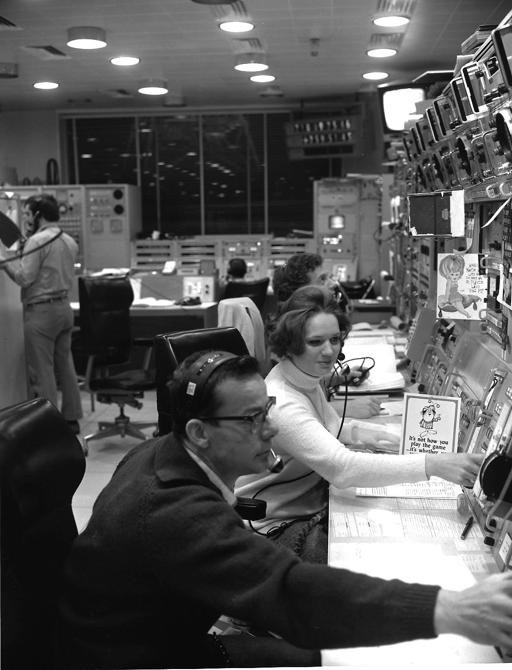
xmin=82 ymin=306 xmax=157 ymax=442
xmin=224 ymin=277 xmax=270 ymax=308
xmin=157 ymin=327 xmax=250 ymax=432
xmin=217 ymin=298 xmax=272 ymax=377
xmin=70 ymin=276 xmax=156 ymax=412
xmin=0 ymin=396 xmax=86 ymax=670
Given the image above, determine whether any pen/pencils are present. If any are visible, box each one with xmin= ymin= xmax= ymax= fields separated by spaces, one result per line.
xmin=459 ymin=515 xmax=475 ymax=544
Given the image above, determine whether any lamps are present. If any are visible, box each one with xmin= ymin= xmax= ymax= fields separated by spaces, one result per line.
xmin=377 ymin=69 xmax=455 ymax=134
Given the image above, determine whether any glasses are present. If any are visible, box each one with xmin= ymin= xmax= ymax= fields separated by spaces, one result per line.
xmin=202 ymin=396 xmax=277 ymax=426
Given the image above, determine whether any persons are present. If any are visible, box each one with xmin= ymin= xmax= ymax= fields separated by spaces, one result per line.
xmin=437 ymin=259 xmax=470 ymax=318
xmin=50 ymin=353 xmax=512 ymax=670
xmin=1 ymin=194 xmax=83 ymax=436
xmin=234 ymin=286 xmax=484 ymax=568
xmin=221 ymin=258 xmax=247 ymax=299
xmin=270 ymin=253 xmax=338 ymax=302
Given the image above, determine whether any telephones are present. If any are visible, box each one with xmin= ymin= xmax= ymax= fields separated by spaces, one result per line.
xmin=174 ymin=297 xmax=201 ymax=305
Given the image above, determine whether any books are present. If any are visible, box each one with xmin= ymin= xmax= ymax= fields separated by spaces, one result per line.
xmin=135 ymin=296 xmax=174 ymax=309
xmin=332 ymin=341 xmax=407 ymax=397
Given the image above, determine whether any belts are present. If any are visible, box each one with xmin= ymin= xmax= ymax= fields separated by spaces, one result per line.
xmin=31 ymin=294 xmax=68 ymax=305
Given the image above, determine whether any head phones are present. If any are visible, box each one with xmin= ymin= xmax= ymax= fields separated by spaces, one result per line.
xmin=176 ymin=350 xmax=284 ymax=475
xmin=28 ymin=193 xmax=47 ymax=226
xmin=280 ymin=284 xmax=351 ymax=377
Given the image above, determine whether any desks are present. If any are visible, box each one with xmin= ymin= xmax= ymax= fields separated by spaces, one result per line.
xmin=70 ymin=302 xmax=217 ymax=326
xmin=322 ymin=324 xmax=512 ymax=666
